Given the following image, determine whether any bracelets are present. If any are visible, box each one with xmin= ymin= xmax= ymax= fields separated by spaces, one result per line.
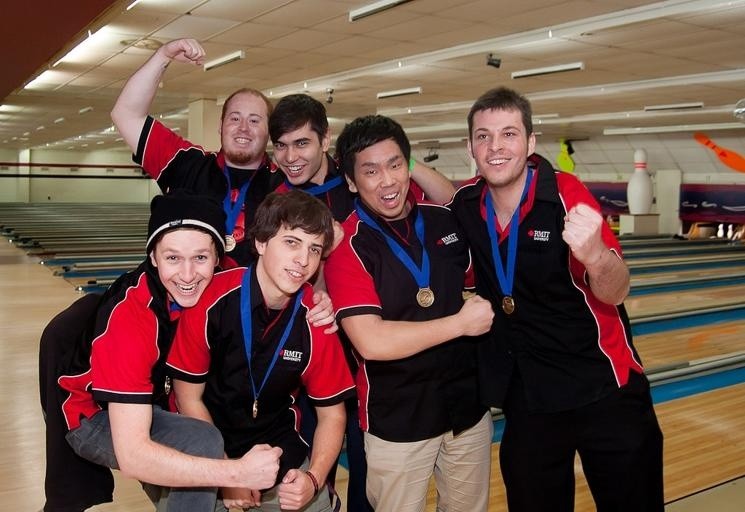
xmin=157 ymin=189 xmax=356 ymax=511
xmin=305 ymin=470 xmax=318 ymax=499
xmin=410 ymin=159 xmax=416 ymax=172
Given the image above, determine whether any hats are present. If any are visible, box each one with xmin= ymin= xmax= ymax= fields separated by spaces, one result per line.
xmin=146 ymin=193 xmax=227 ymax=259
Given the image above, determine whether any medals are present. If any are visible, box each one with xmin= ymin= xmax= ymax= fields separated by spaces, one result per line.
xmin=502 ymin=297 xmax=516 ymax=315
xmin=252 ymin=400 xmax=259 ymax=418
xmin=223 ymin=235 xmax=236 ymax=253
xmin=416 ymin=287 xmax=435 ymax=308
xmin=163 ymin=376 xmax=172 ymax=395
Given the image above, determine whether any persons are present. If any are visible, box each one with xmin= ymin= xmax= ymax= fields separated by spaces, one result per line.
xmin=444 ymin=85 xmax=666 ymax=512
xmin=325 ymin=113 xmax=495 ymax=512
xmin=110 ymin=37 xmax=285 ymax=266
xmin=268 ymin=92 xmax=456 ymax=512
xmin=39 ymin=187 xmax=338 ymax=512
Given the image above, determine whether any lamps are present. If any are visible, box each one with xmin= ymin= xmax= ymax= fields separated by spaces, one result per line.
xmin=376 ymin=87 xmax=423 ymax=99
xmin=644 ymin=102 xmax=703 ymax=111
xmin=203 ymin=48 xmax=245 ymax=72
xmin=348 ymin=0 xmax=413 ymax=23
xmin=510 ymin=62 xmax=585 ymax=80
